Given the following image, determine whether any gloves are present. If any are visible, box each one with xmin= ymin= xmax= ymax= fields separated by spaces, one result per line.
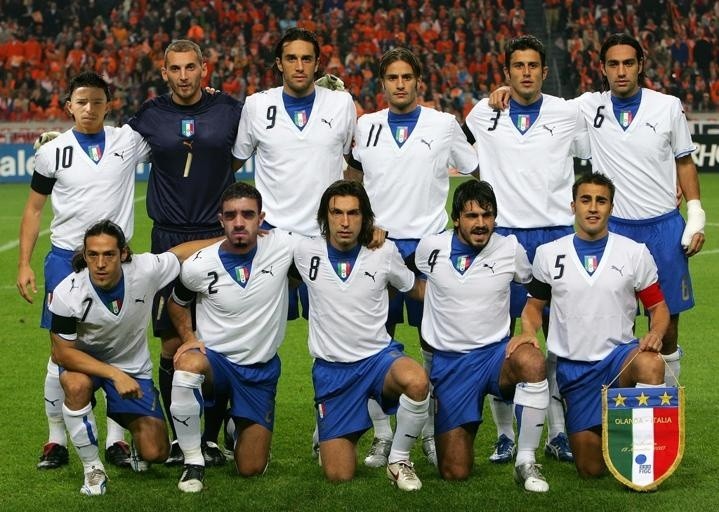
xmin=34 ymin=132 xmax=61 ymax=149
xmin=315 ymin=74 xmax=345 ymax=91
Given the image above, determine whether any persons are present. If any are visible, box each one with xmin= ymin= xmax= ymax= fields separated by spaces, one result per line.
xmin=462 ymin=34 xmax=682 ymax=463
xmin=489 ymin=33 xmax=706 ymax=387
xmin=17 ymin=70 xmax=224 ymax=469
xmin=505 ymin=171 xmax=671 ymax=492
xmin=344 ymin=48 xmax=480 ymax=468
xmin=33 ymin=39 xmax=346 ymax=467
xmin=386 ymin=179 xmax=553 ymax=492
xmin=0 ymin=0 xmax=718 ymax=124
xmin=231 ymin=27 xmax=358 ymax=326
xmin=166 ymin=183 xmax=387 ymax=494
xmin=288 ymin=180 xmax=431 ymax=492
xmin=49 ymin=218 xmax=270 ymax=496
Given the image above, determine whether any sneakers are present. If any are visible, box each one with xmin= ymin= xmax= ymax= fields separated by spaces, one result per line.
xmin=224 ymin=408 xmax=236 ymax=462
xmin=201 ymin=441 xmax=225 ymax=466
xmin=79 ymin=465 xmax=107 ymax=495
xmin=36 ymin=443 xmax=68 ymax=470
xmin=422 ymin=437 xmax=437 ymax=465
xmin=105 ymin=441 xmax=131 ymax=472
xmin=364 ymin=438 xmax=392 ymax=468
xmin=514 ymin=463 xmax=549 ymax=492
xmin=312 ymin=424 xmax=321 ymax=457
xmin=387 ymin=460 xmax=422 ymax=492
xmin=178 ymin=465 xmax=205 ymax=493
xmin=489 ymin=434 xmax=516 ymax=462
xmin=164 ymin=441 xmax=184 ymax=466
xmin=130 ymin=438 xmax=150 ymax=472
xmin=545 ymin=433 xmax=573 ymax=462
